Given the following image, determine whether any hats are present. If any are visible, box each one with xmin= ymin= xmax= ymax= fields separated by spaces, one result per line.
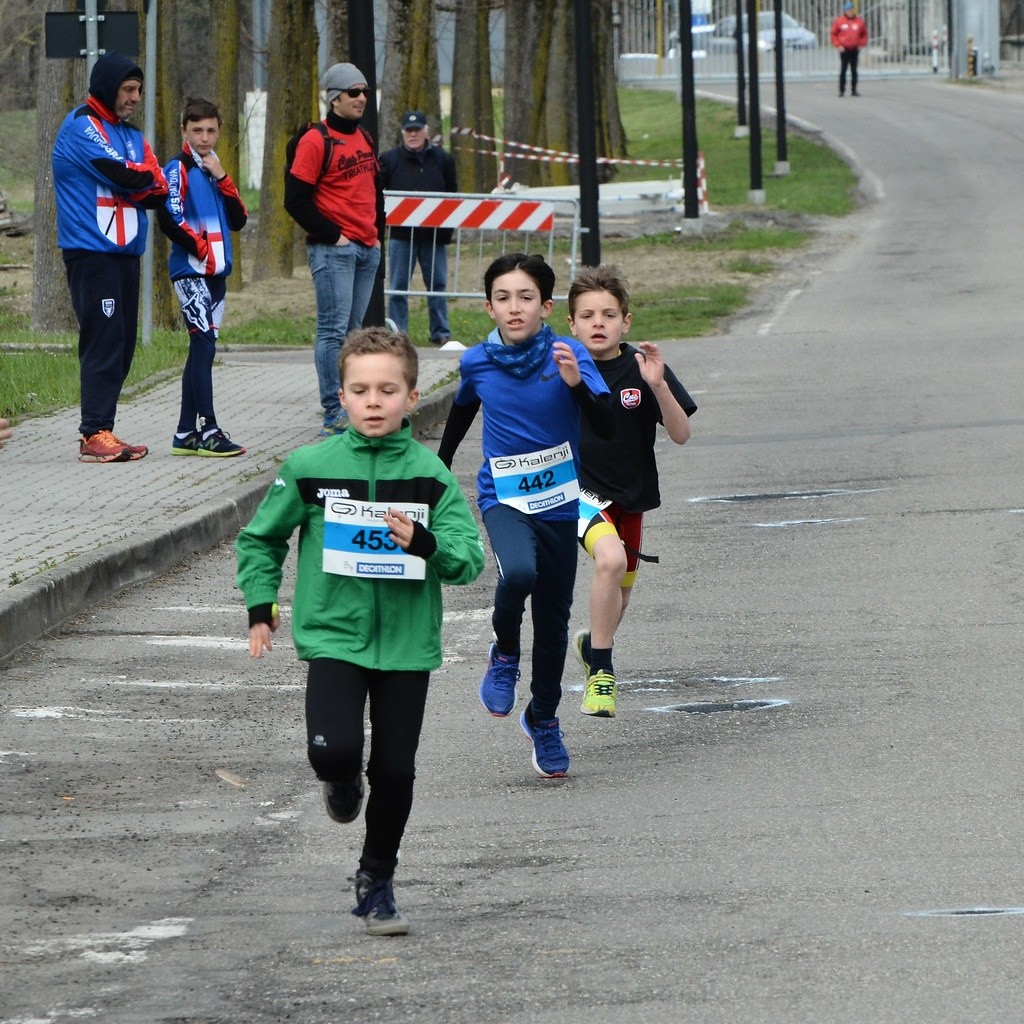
xmin=844 ymin=4 xmax=853 ymax=10
xmin=323 ymin=62 xmax=368 ymax=103
xmin=121 ymin=68 xmax=143 ymax=86
xmin=402 ymin=113 xmax=426 ymax=129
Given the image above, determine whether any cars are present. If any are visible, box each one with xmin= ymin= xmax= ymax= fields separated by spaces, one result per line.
xmin=713 ymin=10 xmax=817 ymax=51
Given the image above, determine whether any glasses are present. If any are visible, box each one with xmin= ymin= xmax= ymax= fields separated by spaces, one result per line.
xmin=327 ymin=88 xmax=371 ymax=98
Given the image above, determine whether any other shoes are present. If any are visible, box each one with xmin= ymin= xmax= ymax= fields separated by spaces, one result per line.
xmin=839 ymin=90 xmax=844 ymax=98
xmin=852 ymin=93 xmax=859 ymax=96
xmin=431 ymin=336 xmax=449 ymax=347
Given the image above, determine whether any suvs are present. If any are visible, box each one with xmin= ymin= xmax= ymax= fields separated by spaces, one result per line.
xmin=668 ymin=23 xmax=716 ymax=58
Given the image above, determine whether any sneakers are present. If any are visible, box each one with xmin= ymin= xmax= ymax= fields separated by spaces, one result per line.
xmin=198 ymin=429 xmax=247 ymax=456
xmin=322 ymin=759 xmax=363 ymax=824
xmin=481 ymin=642 xmax=521 ymax=717
xmin=106 ymin=432 xmax=148 ymax=459
xmin=351 ymin=869 xmax=409 ymax=935
xmin=322 ymin=411 xmax=351 ymax=436
xmin=78 ymin=430 xmax=131 ymax=462
xmin=519 ymin=710 xmax=568 ymax=777
xmin=574 ymin=628 xmax=595 ymax=673
xmin=170 ymin=430 xmax=203 ymax=454
xmin=580 ymin=668 xmax=617 ymax=717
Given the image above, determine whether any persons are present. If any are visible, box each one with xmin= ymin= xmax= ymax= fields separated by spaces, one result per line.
xmin=52 ymin=55 xmax=170 ymax=463
xmin=831 ymin=3 xmax=867 ymax=96
xmin=286 ymin=64 xmax=386 ymax=438
xmin=235 ymin=329 xmax=485 ymax=933
xmin=379 ymin=112 xmax=457 ymax=344
xmin=567 ymin=272 xmax=698 ymax=717
xmin=437 ymin=253 xmax=615 ymax=777
xmin=159 ymin=100 xmax=249 ymax=457
